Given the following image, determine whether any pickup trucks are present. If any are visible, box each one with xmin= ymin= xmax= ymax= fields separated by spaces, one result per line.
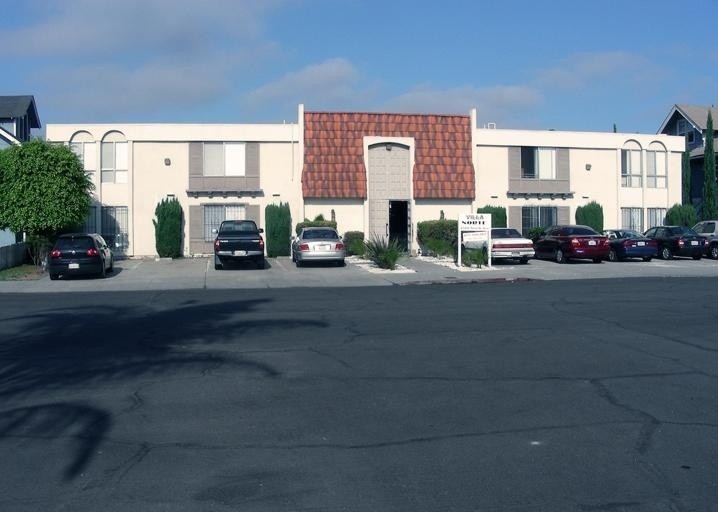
xmin=214 ymin=220 xmax=264 ymax=270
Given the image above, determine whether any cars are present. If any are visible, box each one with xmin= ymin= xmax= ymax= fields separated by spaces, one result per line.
xmin=48 ymin=233 xmax=114 ymax=280
xmin=452 ymin=220 xmax=718 ymax=264
xmin=290 ymin=227 xmax=346 ymax=267
